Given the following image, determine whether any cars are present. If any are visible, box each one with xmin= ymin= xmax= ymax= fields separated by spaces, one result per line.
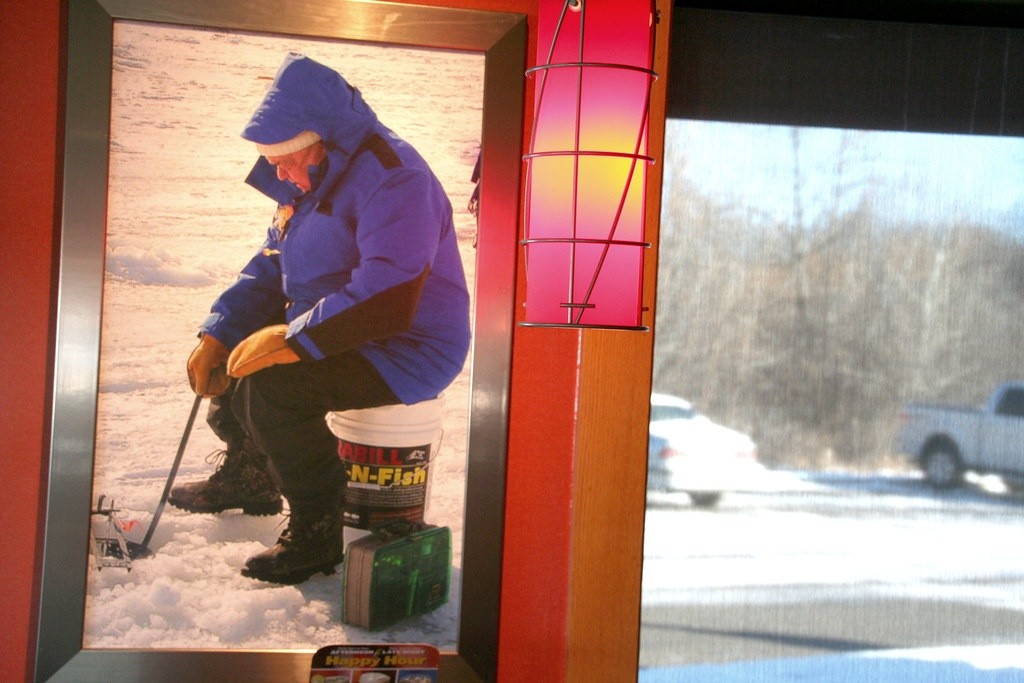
xmin=903 ymin=380 xmax=1024 ymax=489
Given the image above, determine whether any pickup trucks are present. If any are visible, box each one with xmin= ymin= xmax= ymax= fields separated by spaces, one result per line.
xmin=644 ymin=393 xmax=754 ymax=509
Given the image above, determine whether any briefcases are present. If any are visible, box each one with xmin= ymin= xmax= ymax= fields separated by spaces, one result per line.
xmin=341 ymin=517 xmax=451 ymax=631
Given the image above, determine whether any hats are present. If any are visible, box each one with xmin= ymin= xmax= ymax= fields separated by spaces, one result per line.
xmin=254 ymin=130 xmax=322 ymax=157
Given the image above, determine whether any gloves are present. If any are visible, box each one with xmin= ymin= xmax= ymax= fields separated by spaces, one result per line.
xmin=226 ymin=322 xmax=301 ymax=378
xmin=185 ymin=334 xmax=234 ymax=397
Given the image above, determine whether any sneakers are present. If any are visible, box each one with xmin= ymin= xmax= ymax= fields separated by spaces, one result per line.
xmin=240 ymin=504 xmax=344 ymax=585
xmin=168 ymin=437 xmax=283 ymax=518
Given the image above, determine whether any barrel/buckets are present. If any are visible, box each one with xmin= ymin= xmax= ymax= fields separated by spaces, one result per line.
xmin=322 ymin=397 xmax=445 ymax=531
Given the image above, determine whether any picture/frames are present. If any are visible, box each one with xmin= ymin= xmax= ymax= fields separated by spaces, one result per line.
xmin=29 ymin=0 xmax=531 ymax=683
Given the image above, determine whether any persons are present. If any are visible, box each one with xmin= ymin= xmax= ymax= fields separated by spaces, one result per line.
xmin=166 ymin=51 xmax=472 ymax=586
